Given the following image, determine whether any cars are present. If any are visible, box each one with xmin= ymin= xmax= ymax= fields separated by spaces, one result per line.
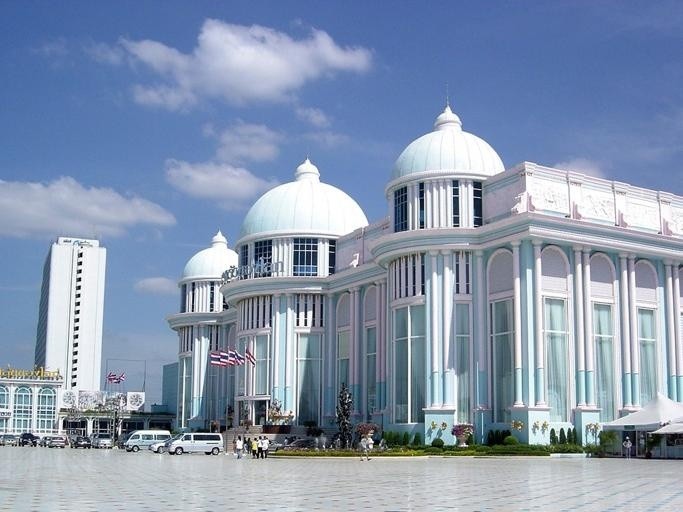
xmin=0 ymin=435 xmax=17 ymax=447
xmin=40 ymin=435 xmax=65 ymax=448
xmin=19 ymin=433 xmax=36 ymax=447
xmin=70 ymin=436 xmax=91 ymax=448
xmin=93 ymin=433 xmax=112 ymax=449
xmin=151 ymin=437 xmax=174 ymax=452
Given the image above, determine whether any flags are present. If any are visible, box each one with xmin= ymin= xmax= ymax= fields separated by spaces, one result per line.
xmin=209 ymin=352 xmax=220 ymax=367
xmin=106 ymin=370 xmax=125 ymax=383
xmin=233 ymin=349 xmax=244 ymax=366
xmin=219 ymin=350 xmax=230 ymax=367
xmin=228 ymin=347 xmax=235 ymax=366
xmin=245 ymin=347 xmax=256 ymax=367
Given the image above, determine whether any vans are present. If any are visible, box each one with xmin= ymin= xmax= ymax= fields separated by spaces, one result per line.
xmin=120 ymin=429 xmax=171 ymax=452
xmin=168 ymin=432 xmax=225 ymax=455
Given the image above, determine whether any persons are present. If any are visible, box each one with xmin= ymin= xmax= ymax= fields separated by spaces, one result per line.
xmin=233 ymin=431 xmax=301 ymax=459
xmin=359 ymin=433 xmax=371 ymax=461
xmin=622 ymin=435 xmax=632 ymax=458
